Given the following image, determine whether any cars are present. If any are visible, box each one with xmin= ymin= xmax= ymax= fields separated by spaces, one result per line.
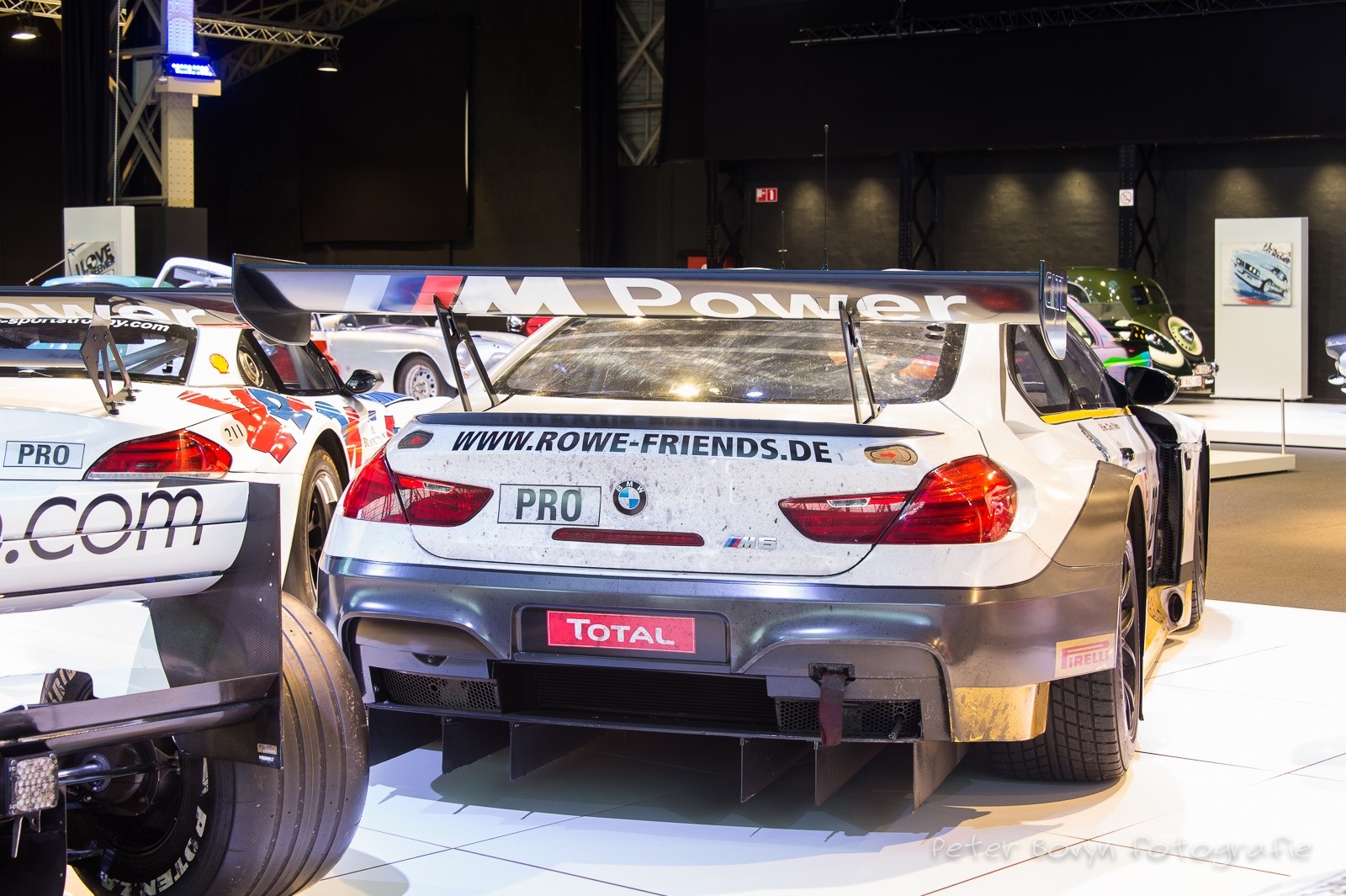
xmin=1 ymin=253 xmax=1220 ymax=895
